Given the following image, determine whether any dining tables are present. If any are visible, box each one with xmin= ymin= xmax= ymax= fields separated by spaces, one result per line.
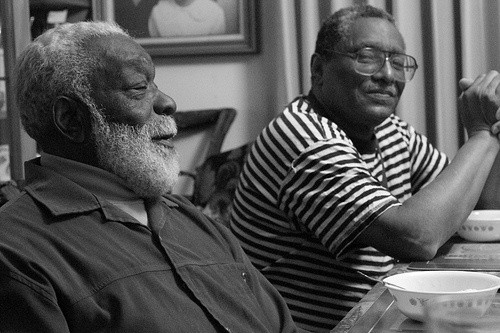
xmin=328 ymin=224 xmax=500 ymax=333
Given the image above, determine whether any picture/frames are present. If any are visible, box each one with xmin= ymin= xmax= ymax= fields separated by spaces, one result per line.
xmin=102 ymin=0 xmax=259 ymax=57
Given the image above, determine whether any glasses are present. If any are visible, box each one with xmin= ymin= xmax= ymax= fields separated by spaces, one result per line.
xmin=320 ymin=46 xmax=418 ymax=83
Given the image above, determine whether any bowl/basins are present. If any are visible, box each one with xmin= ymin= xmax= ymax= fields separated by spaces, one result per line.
xmin=457 ymin=209 xmax=500 ymax=242
xmin=383 ymin=271 xmax=500 ymax=322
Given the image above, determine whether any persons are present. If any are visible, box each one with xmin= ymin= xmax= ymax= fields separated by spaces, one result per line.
xmin=227 ymin=4 xmax=500 ymax=333
xmin=113 ymin=0 xmax=240 ymax=37
xmin=0 ymin=20 xmax=311 ymax=333
